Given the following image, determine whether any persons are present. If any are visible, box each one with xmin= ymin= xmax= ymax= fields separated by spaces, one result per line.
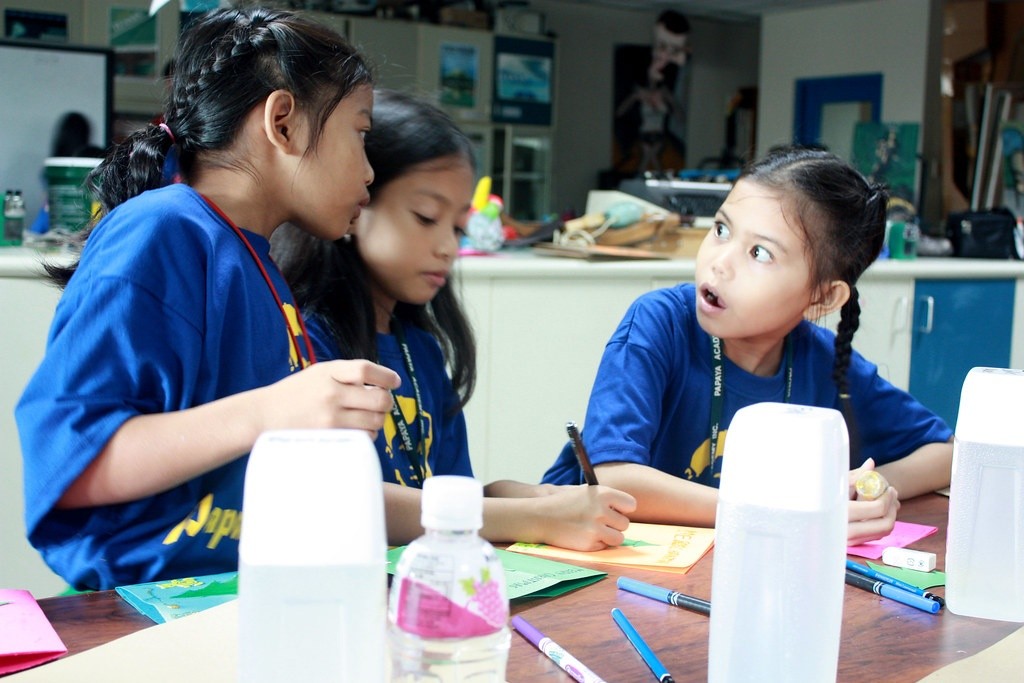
xmin=50 ymin=110 xmax=111 ymax=159
xmin=539 ymin=150 xmax=956 ymax=550
xmin=594 ymin=130 xmax=686 ymax=197
xmin=14 ymin=9 xmax=403 ymax=596
xmin=266 ymin=84 xmax=637 ymax=554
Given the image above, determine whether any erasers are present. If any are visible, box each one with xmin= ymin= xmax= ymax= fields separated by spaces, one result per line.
xmin=881 ymin=546 xmax=937 ymax=573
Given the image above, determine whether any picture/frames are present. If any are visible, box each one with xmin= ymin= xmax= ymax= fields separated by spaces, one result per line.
xmin=488 ymin=35 xmax=556 ymax=128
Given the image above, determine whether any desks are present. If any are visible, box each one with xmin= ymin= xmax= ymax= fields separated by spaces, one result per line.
xmin=0 ymin=499 xmax=1024 ymax=683
xmin=645 ymin=179 xmax=733 ymax=218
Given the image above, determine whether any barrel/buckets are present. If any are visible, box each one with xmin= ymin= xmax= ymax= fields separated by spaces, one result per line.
xmin=42 ymin=155 xmax=104 ymax=232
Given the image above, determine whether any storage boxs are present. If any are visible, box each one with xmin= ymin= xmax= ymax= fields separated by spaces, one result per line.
xmin=440 ymin=6 xmax=489 ymax=29
xmin=493 ymin=8 xmax=546 ymax=35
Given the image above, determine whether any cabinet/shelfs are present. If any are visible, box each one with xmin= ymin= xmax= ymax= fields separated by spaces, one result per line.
xmin=0 ymin=247 xmax=1024 ymax=603
xmin=0 ymin=0 xmax=179 ymax=116
xmin=491 ymin=124 xmax=554 ymax=222
xmin=304 ymin=10 xmax=417 ymax=99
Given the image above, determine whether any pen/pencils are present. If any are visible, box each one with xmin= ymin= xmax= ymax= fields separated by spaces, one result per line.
xmin=510 ymin=615 xmax=608 ymax=683
xmin=846 ymin=560 xmax=946 ymax=608
xmin=610 ymin=606 xmax=675 ymax=683
xmin=567 ymin=422 xmax=599 ymax=485
xmin=616 ymin=576 xmax=711 ymax=617
xmin=844 ymin=572 xmax=942 ymax=614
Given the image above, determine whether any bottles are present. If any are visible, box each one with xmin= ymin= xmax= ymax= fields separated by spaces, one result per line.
xmin=2 ymin=190 xmax=25 ymax=238
xmin=385 ymin=476 xmax=512 ymax=683
